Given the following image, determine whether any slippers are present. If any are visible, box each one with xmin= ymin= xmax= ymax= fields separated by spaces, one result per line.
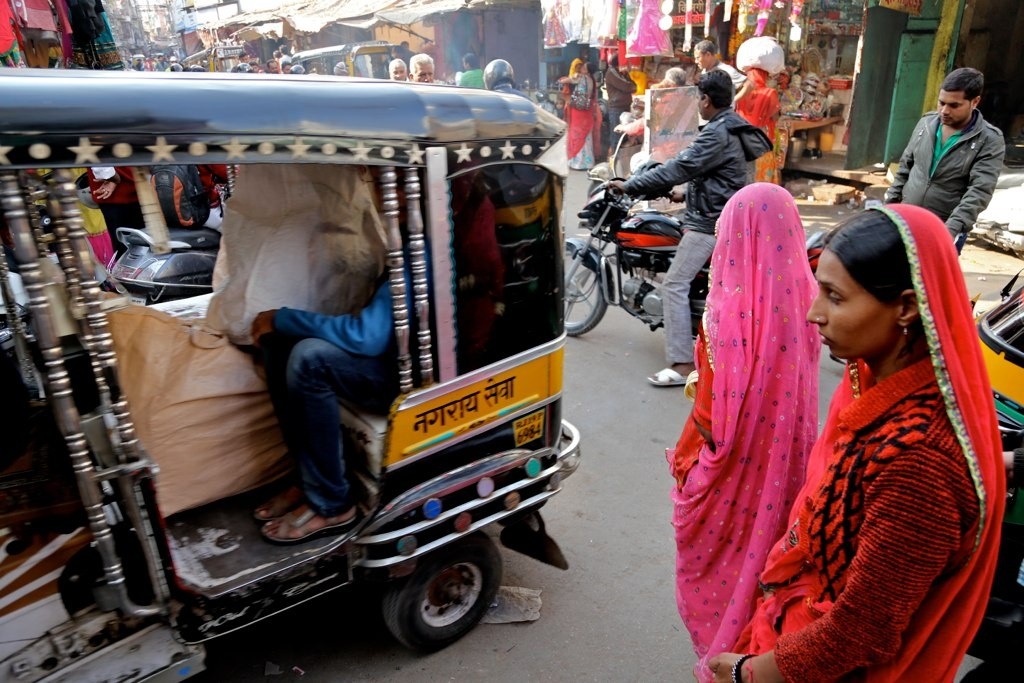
xmin=260 ymin=506 xmax=357 ymax=543
xmin=647 ymin=366 xmax=692 ymax=387
xmin=255 ymin=491 xmax=302 ymax=522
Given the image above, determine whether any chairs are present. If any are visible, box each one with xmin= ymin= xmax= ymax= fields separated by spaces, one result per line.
xmin=332 ymin=396 xmax=388 ymax=518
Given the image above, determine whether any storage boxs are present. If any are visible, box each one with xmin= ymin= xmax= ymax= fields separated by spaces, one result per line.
xmin=829 ymin=79 xmax=853 ymax=90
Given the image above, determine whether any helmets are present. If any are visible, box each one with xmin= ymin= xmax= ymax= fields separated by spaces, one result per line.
xmin=72 ymin=170 xmax=99 ymax=209
xmin=183 ymin=64 xmax=206 ymax=72
xmin=232 ymin=63 xmax=253 ymax=73
xmin=171 ymin=63 xmax=182 ymax=72
xmin=484 ymin=58 xmax=516 ymax=88
xmin=290 ymin=65 xmax=305 ymax=74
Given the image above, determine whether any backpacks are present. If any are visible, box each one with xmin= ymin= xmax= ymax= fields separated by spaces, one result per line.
xmin=150 ymin=162 xmax=210 ymax=231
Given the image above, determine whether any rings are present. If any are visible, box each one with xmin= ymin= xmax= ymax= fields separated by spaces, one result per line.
xmin=103 ymin=189 xmax=105 ymax=192
xmin=105 ymin=191 xmax=108 ymax=194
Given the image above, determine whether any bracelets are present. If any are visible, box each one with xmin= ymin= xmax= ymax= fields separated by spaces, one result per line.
xmin=731 ymin=653 xmax=759 ymax=683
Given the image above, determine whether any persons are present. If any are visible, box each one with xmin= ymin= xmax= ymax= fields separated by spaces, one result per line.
xmin=87 ymin=163 xmax=240 ymax=260
xmin=605 ymin=71 xmax=773 ymax=387
xmin=543 ymin=52 xmax=637 ymax=171
xmin=136 ymin=55 xmax=207 ymax=73
xmin=667 ymin=182 xmax=822 ymax=683
xmin=705 ymin=201 xmax=1006 ymax=683
xmin=230 ymin=45 xmax=350 ymax=76
xmin=829 ymin=68 xmax=1006 ymax=365
xmin=388 ymin=41 xmax=533 ymax=102
xmin=251 ymin=172 xmax=438 ymax=548
xmin=613 ymin=40 xmax=755 ymax=164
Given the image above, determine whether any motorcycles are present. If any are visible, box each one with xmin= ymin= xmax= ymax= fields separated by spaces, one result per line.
xmin=563 ymin=115 xmax=835 ymax=355
xmin=966 ymin=232 xmax=1024 ymax=671
xmin=0 ymin=64 xmax=581 ymax=683
xmin=347 ymin=44 xmax=399 ymax=79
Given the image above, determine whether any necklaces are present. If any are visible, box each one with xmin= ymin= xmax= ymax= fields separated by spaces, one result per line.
xmin=847 ymin=359 xmax=861 ymax=399
